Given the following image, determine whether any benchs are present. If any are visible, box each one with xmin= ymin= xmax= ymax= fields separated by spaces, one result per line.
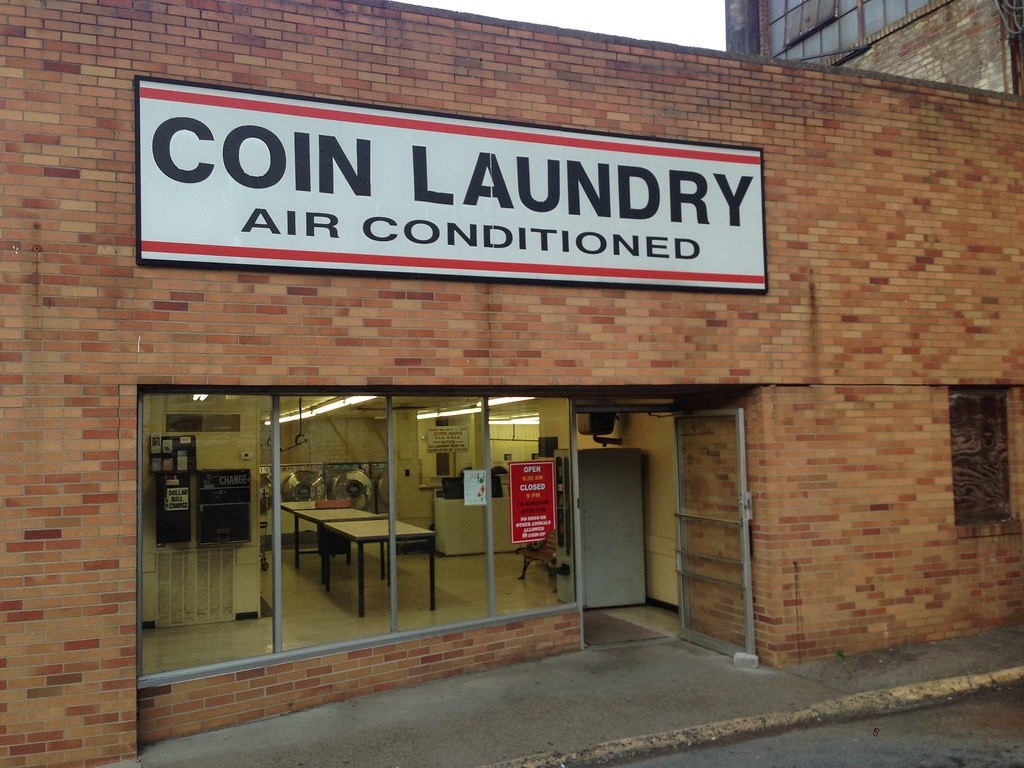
xmin=514 ymin=508 xmax=562 ymax=595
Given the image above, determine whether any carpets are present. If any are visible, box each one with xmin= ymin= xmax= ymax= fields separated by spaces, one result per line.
xmin=582 ymin=608 xmax=670 ymax=646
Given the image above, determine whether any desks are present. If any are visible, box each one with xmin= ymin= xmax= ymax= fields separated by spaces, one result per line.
xmin=293 ymin=507 xmax=387 ymax=587
xmin=323 ymin=519 xmax=437 ymax=618
xmin=279 ymin=500 xmax=337 ymax=558
